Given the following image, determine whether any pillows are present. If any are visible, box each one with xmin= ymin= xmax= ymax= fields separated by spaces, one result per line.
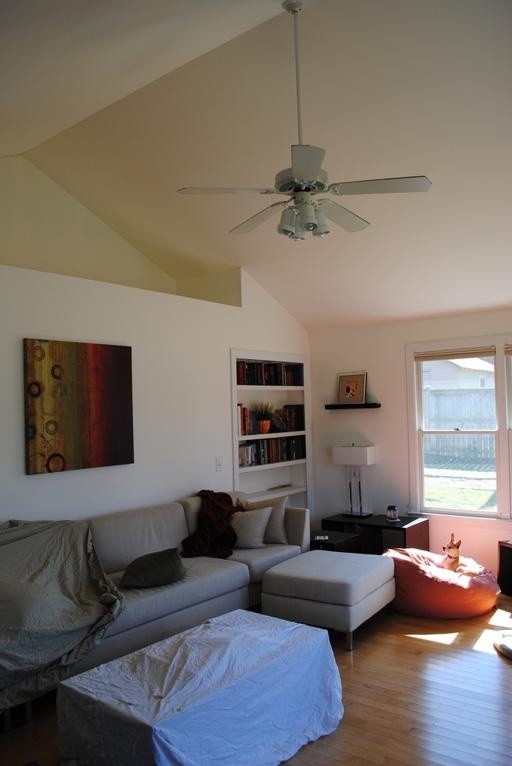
xmin=232 ymin=506 xmax=274 ymax=549
xmin=236 ymin=495 xmax=289 ymax=544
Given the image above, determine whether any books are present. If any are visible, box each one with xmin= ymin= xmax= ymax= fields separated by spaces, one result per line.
xmin=237 ymin=361 xmax=303 ymax=386
xmin=237 ymin=403 xmax=258 ymax=435
xmin=271 ymin=404 xmax=304 ymax=432
xmin=239 ymin=435 xmax=306 ymax=467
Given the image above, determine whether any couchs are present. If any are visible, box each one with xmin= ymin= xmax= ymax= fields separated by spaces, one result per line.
xmin=261 ymin=548 xmax=397 ymax=650
xmin=381 ymin=546 xmax=502 ymax=618
xmin=0 ymin=491 xmax=311 ymax=719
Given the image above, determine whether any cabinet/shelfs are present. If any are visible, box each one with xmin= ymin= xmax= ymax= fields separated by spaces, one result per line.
xmin=320 ymin=512 xmax=430 ymax=555
xmin=230 ymin=348 xmax=317 ymax=533
xmin=497 ymin=539 xmax=512 ymax=597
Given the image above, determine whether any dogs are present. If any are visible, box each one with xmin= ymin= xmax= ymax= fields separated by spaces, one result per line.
xmin=437 ymin=533 xmax=467 ymax=573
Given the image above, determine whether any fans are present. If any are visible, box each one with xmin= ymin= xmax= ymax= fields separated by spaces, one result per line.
xmin=175 ymin=0 xmax=432 ymax=233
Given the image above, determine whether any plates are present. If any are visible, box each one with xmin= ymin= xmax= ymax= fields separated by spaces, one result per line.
xmin=387 ymin=518 xmax=400 ymax=523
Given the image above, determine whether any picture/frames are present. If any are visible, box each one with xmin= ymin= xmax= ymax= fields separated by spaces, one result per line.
xmin=334 ymin=371 xmax=368 ymax=404
xmin=23 ymin=336 xmax=134 ymax=471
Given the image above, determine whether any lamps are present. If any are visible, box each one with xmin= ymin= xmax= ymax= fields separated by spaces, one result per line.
xmin=289 ymin=212 xmax=306 ymax=242
xmin=331 ymin=441 xmax=379 ymax=518
xmin=277 ymin=205 xmax=297 ymax=236
xmin=298 ymin=201 xmax=318 ymax=231
xmin=312 ymin=207 xmax=330 ymax=239
